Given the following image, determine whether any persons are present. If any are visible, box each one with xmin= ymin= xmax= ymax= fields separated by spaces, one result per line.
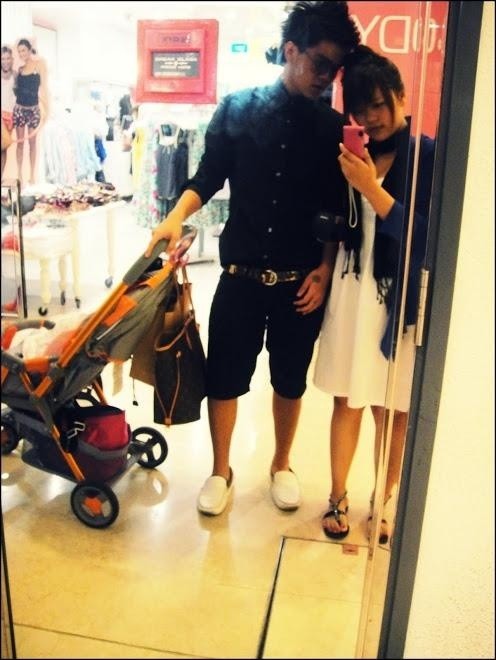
xmin=99 ymin=93 xmax=135 ymax=204
xmin=319 ymin=41 xmax=435 ymax=544
xmin=0 ymin=46 xmax=17 ymax=182
xmin=138 ymin=1 xmax=364 ymax=519
xmin=11 ymin=38 xmax=51 ymax=188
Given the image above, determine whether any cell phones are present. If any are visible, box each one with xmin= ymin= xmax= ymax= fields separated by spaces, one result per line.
xmin=343 ymin=126 xmax=365 ymax=162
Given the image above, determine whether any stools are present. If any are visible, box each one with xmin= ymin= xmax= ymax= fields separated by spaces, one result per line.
xmin=0 ymin=221 xmax=72 ymax=318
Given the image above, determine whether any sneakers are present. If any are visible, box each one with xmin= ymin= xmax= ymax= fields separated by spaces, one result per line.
xmin=197 ymin=466 xmax=235 ymax=516
xmin=270 ymin=467 xmax=303 ymax=511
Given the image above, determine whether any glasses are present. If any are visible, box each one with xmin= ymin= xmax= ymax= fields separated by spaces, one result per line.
xmin=300 ymin=46 xmax=340 ymax=75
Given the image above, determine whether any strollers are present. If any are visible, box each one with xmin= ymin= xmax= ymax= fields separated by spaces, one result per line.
xmin=1 ymin=226 xmax=202 ymax=528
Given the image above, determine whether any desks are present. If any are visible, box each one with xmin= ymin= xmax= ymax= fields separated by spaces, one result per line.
xmin=1 ymin=195 xmax=135 ymax=316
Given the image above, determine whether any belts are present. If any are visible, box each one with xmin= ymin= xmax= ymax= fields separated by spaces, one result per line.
xmin=223 ymin=264 xmax=310 ymax=287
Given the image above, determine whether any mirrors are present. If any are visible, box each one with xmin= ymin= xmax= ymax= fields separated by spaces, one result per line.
xmin=0 ymin=1 xmax=488 ymax=660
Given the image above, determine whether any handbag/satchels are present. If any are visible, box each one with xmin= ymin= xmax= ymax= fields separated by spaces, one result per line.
xmin=152 ymin=263 xmax=207 ymax=427
xmin=128 ymin=266 xmax=193 ymax=387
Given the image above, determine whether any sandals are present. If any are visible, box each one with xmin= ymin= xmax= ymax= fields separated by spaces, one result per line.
xmin=322 ymin=491 xmax=349 ymax=540
xmin=367 ymin=494 xmax=393 ymax=545
